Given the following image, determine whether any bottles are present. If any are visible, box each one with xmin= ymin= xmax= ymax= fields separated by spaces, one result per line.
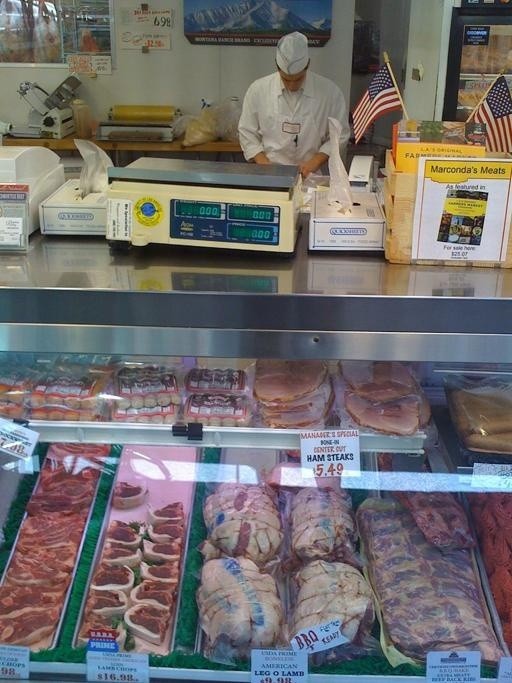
xmin=73 ymin=98 xmax=90 ymax=139
xmin=230 ymin=96 xmax=242 ymax=127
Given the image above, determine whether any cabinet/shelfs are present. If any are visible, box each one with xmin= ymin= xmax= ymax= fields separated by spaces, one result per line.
xmin=1 ymin=351 xmax=511 ymax=682
xmin=443 ymin=6 xmax=512 ymax=121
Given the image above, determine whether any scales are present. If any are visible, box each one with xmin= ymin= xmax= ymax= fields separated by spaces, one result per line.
xmin=106 ymin=156 xmax=303 ymax=258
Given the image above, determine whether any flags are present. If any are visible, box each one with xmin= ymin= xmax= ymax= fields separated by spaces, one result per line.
xmin=350 ymin=62 xmax=403 ymax=145
xmin=471 ymin=74 xmax=512 ymax=155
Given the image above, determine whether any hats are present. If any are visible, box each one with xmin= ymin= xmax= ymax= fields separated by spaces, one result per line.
xmin=274 ymin=30 xmax=310 ymax=76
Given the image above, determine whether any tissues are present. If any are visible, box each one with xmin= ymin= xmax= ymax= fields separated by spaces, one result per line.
xmin=36 ymin=133 xmax=113 ymax=236
xmin=306 ymin=114 xmax=386 ymax=254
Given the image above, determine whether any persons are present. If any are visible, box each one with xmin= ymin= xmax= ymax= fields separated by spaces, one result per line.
xmin=238 ymin=30 xmax=350 ymax=183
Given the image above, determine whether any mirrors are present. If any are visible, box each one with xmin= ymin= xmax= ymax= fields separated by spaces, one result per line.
xmin=0 ymin=1 xmax=117 ymax=69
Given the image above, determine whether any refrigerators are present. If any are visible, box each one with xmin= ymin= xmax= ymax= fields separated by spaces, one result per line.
xmin=402 ymin=0 xmax=512 ymax=123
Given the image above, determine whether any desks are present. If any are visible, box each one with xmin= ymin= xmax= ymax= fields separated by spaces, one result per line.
xmin=1 ymin=136 xmax=256 ymax=166
